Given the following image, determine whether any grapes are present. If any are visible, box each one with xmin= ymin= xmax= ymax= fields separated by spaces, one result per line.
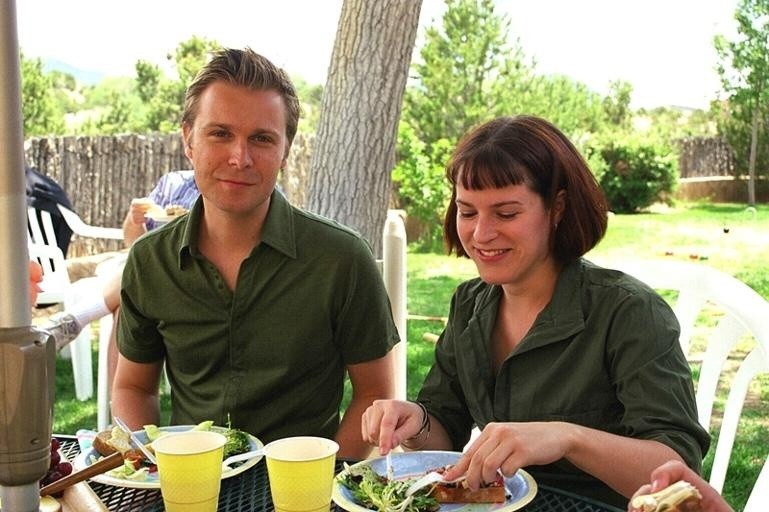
xmin=37 ymin=438 xmax=72 ymax=498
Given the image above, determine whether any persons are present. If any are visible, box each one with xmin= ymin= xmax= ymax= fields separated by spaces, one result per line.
xmin=109 ymin=44 xmax=401 ymax=464
xmin=359 ymin=113 xmax=711 ymax=500
xmin=626 ymin=459 xmax=738 ymax=512
xmin=26 ymin=167 xmax=202 ymax=387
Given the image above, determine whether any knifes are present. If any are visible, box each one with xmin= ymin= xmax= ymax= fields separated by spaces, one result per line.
xmin=112 ymin=414 xmax=159 ymax=467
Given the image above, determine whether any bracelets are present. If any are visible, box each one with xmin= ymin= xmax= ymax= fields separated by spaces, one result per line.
xmin=408 ymin=399 xmax=428 ymax=440
xmin=398 ymin=418 xmax=432 ymax=451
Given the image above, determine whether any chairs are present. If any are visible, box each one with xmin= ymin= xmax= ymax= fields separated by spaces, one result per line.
xmin=25 ymin=199 xmax=125 ymax=400
xmin=93 ymin=246 xmax=173 ymax=435
xmin=599 ymin=255 xmax=767 ymax=511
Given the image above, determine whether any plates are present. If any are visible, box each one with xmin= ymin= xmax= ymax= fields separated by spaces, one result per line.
xmin=330 ymin=449 xmax=540 ymax=511
xmin=72 ymin=424 xmax=265 ymax=489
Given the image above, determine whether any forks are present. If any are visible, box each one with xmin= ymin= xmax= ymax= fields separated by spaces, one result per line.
xmin=404 ymin=470 xmax=467 ymax=497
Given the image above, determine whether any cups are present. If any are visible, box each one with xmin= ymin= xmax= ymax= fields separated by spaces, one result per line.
xmin=150 ymin=430 xmax=228 ymax=512
xmin=262 ymin=434 xmax=340 ymax=512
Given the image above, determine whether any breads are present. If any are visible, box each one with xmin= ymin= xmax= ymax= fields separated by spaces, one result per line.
xmin=424 ymin=464 xmax=504 ymax=503
xmin=94 ymin=431 xmax=131 ymax=457
xmin=631 ymin=481 xmax=703 ymax=512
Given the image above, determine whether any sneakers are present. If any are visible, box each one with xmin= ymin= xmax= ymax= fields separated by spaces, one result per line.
xmin=30 ymin=311 xmax=83 ymax=355
